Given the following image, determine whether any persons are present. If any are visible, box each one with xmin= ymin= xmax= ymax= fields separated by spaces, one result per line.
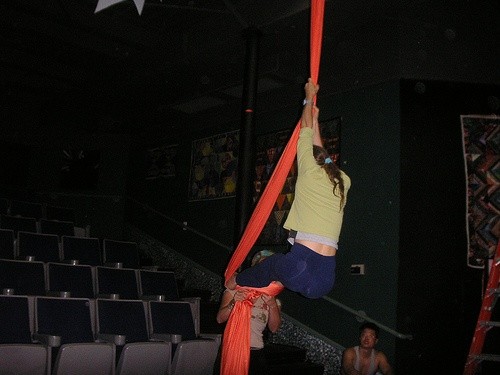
xmin=213 ymin=249 xmax=282 ymax=375
xmin=340 ymin=322 xmax=394 ymax=375
xmin=224 ymin=78 xmax=352 ymax=300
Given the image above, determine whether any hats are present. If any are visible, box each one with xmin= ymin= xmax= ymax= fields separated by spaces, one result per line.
xmin=251 ymin=250 xmax=274 ymax=267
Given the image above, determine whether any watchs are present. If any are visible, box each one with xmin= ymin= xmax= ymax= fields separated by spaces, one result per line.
xmin=302 ymin=99 xmax=312 ymax=105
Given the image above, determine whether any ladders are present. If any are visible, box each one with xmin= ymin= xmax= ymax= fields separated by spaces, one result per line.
xmin=463 ymin=242 xmax=500 ymax=375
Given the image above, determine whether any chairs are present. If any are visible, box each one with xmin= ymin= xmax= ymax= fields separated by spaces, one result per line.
xmin=0 ymin=198 xmax=223 ymax=375
